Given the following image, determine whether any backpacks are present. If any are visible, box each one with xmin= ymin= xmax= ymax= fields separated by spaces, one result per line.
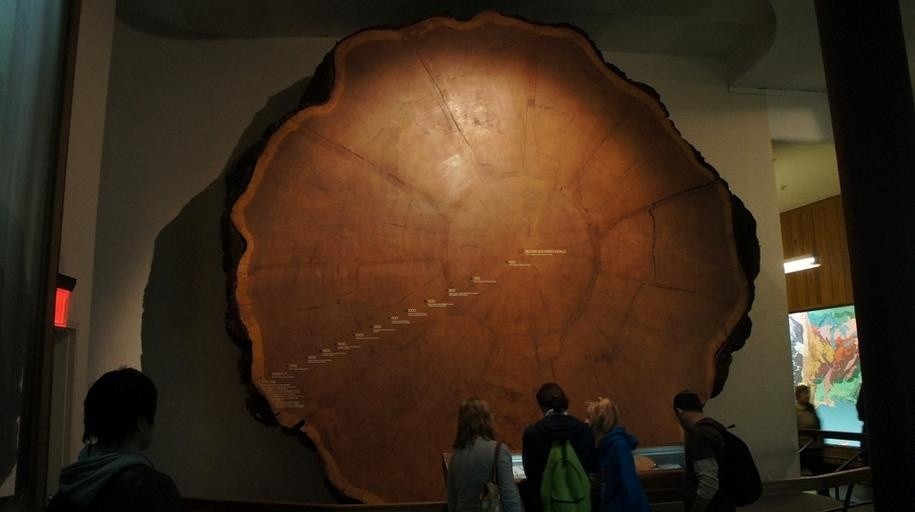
xmin=534 ymin=420 xmax=593 ymax=512
xmin=695 ymin=421 xmax=764 ymax=508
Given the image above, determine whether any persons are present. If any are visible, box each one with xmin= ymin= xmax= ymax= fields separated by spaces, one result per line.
xmin=795 ymin=385 xmax=830 ymax=499
xmin=447 ymin=397 xmax=523 ymax=512
xmin=674 ymin=390 xmax=736 ymax=512
xmin=524 ymin=382 xmax=649 ymax=512
xmin=45 ymin=368 xmax=184 ymax=512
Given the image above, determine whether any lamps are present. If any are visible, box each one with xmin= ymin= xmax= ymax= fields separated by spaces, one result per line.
xmin=784 ymin=253 xmax=822 ymax=275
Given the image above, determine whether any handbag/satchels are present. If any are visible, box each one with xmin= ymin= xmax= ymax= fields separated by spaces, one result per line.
xmin=480 ymin=479 xmax=505 ymax=512
